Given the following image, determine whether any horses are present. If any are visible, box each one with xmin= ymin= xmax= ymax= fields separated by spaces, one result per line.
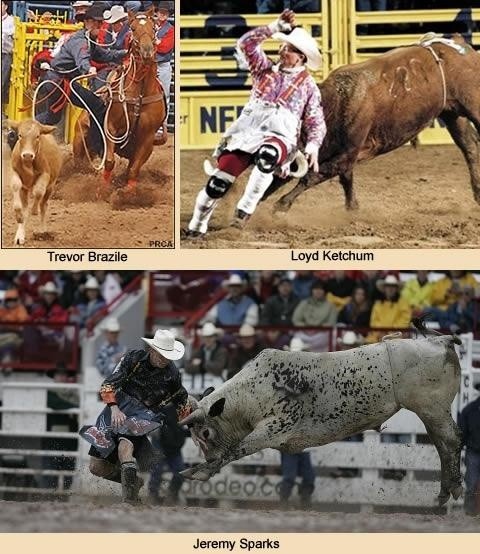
xmin=74 ymin=4 xmax=167 ymax=202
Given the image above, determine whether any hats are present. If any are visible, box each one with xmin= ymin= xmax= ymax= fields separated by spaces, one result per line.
xmin=100 ymin=317 xmax=120 ymax=331
xmin=140 ymin=329 xmax=185 ymax=361
xmin=196 ymin=322 xmax=255 ymax=337
xmin=376 ymin=274 xmax=405 ymax=292
xmin=226 ymin=274 xmax=243 ymax=286
xmin=278 ymin=277 xmax=327 ymax=291
xmin=72 ymin=0 xmax=128 ymax=24
xmin=271 ymin=27 xmax=322 ymax=71
xmin=85 ymin=276 xmax=100 ymax=289
xmin=38 ymin=281 xmax=59 ymax=294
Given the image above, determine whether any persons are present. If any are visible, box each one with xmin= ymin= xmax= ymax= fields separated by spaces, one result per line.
xmin=0 ymin=270 xmax=480 ymax=522
xmin=187 ymin=7 xmax=328 ymax=237
xmin=1 ymin=1 xmax=175 ymax=170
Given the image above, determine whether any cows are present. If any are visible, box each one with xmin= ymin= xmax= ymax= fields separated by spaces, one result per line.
xmin=267 ymin=30 xmax=480 ymax=220
xmin=176 ymin=310 xmax=467 ymax=509
xmin=3 ymin=116 xmax=63 ymax=246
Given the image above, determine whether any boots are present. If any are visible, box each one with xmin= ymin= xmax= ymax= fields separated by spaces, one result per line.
xmin=122 ymin=462 xmax=145 ymax=500
